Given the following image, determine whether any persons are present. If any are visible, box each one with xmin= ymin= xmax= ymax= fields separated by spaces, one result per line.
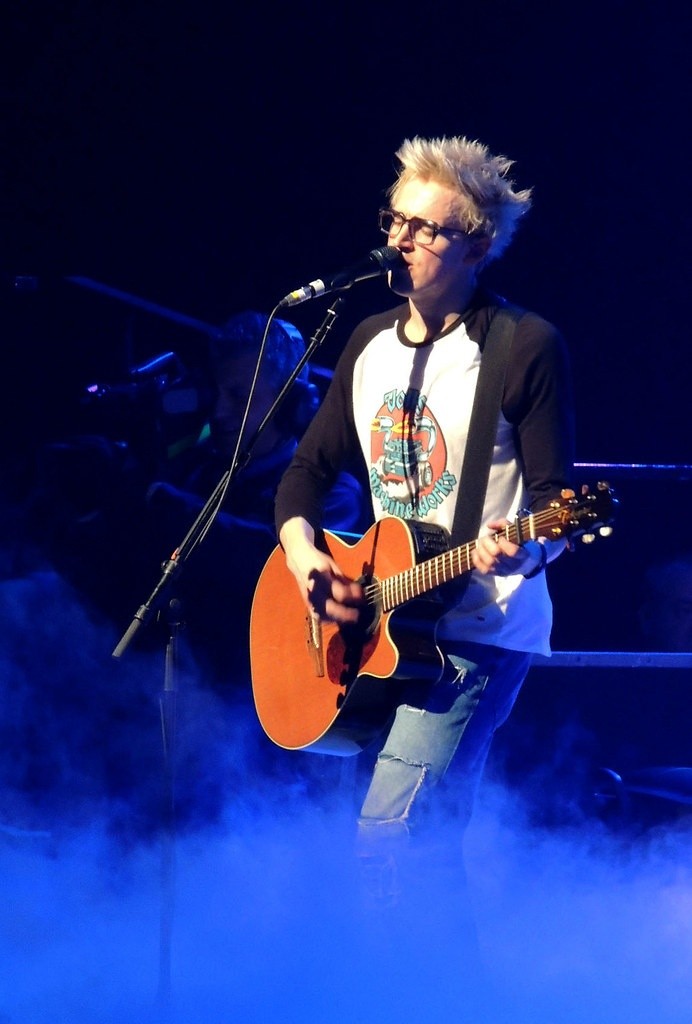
xmin=144 ymin=310 xmax=370 ymax=832
xmin=274 ymin=134 xmax=579 ymax=1022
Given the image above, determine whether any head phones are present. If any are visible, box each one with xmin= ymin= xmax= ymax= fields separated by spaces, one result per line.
xmin=209 ymin=313 xmax=320 ymax=440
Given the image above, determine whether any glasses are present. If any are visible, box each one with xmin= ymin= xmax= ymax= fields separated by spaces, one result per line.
xmin=378 ymin=207 xmax=469 ymax=246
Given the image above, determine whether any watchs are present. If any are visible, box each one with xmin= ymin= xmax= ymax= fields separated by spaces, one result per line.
xmin=523 ymin=542 xmax=548 ymax=581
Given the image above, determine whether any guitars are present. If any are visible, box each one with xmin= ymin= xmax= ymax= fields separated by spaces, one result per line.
xmin=250 ymin=481 xmax=622 ymax=758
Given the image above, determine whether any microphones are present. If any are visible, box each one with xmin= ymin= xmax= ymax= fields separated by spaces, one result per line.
xmin=279 ymin=244 xmax=401 ymax=308
